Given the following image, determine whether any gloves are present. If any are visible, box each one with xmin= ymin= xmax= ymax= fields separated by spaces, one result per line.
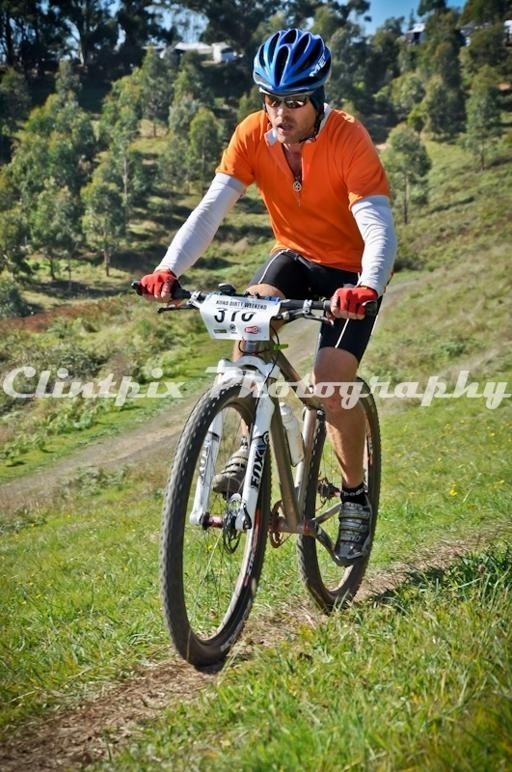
xmin=138 ymin=269 xmax=179 ymax=298
xmin=330 ymin=284 xmax=378 ymax=316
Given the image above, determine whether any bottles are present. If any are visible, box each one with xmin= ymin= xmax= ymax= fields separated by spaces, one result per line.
xmin=278 ymin=400 xmax=305 ymax=467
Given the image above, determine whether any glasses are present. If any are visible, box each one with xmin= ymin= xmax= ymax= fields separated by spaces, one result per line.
xmin=262 ymin=95 xmax=308 ymax=109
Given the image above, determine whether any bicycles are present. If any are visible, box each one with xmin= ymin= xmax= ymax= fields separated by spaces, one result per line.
xmin=131 ymin=280 xmax=381 ymax=667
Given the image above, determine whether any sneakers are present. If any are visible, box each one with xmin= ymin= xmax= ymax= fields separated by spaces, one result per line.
xmin=211 ymin=436 xmax=251 ymax=494
xmin=333 ymin=484 xmax=372 ymax=560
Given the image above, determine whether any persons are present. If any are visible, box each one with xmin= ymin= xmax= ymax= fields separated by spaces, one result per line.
xmin=142 ymin=28 xmax=397 ymax=566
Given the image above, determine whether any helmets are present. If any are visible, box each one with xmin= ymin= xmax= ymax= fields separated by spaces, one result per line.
xmin=252 ymin=28 xmax=332 ymax=98
